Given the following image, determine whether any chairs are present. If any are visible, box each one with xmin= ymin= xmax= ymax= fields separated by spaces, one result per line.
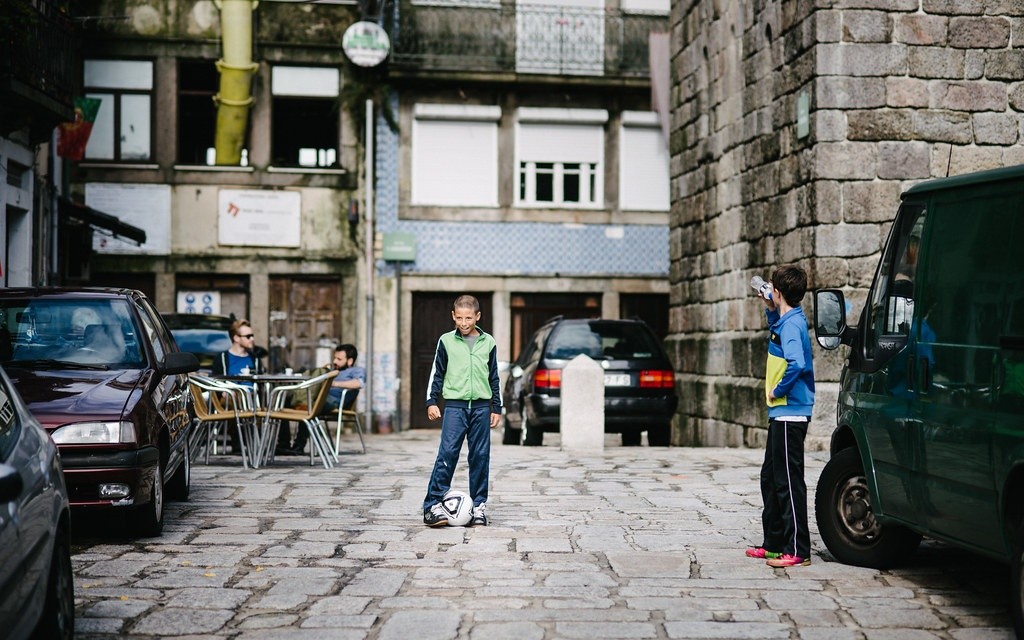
xmin=186 ymin=370 xmax=368 ymax=470
xmin=83 ymin=324 xmax=133 ymax=362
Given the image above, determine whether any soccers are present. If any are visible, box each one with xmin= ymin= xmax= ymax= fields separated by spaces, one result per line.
xmin=441 ymin=491 xmax=473 ymax=526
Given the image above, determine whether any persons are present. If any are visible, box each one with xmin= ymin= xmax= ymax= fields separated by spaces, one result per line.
xmin=745 ymin=265 xmax=815 ymax=568
xmin=275 ymin=344 xmax=367 ymax=456
xmin=423 ymin=295 xmax=504 ymax=529
xmin=211 ymin=319 xmax=268 ymax=456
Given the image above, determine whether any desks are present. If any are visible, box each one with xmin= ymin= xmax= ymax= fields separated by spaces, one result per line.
xmin=215 ymin=376 xmax=315 ymax=467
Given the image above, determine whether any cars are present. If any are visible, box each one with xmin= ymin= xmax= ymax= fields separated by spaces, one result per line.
xmin=157 ymin=313 xmax=268 ymax=419
xmin=502 ymin=315 xmax=679 ymax=446
xmin=0 ymin=368 xmax=76 ymax=639
xmin=0 ymin=288 xmax=200 ymax=536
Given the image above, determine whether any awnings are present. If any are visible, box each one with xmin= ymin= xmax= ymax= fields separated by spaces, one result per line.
xmin=60 ymin=198 xmax=147 ymax=248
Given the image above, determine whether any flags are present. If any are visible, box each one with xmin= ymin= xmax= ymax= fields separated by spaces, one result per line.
xmin=56 ymin=97 xmax=102 ymax=163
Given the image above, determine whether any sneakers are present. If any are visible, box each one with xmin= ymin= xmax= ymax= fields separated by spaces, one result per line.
xmin=470 ymin=502 xmax=487 ymax=526
xmin=745 ymin=546 xmax=783 ymax=559
xmin=766 ymin=553 xmax=811 ymax=567
xmin=424 ymin=502 xmax=448 ymax=526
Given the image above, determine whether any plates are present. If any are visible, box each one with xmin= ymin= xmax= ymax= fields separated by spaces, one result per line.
xmin=239 ymin=374 xmax=253 ymax=376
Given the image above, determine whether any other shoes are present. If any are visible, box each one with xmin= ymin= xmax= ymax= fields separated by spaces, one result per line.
xmin=265 ymin=444 xmax=291 ymax=455
xmin=291 ymin=444 xmax=303 ymax=454
xmin=232 ymin=447 xmax=241 ymax=454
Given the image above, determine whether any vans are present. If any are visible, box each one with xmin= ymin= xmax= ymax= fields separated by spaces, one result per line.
xmin=812 ymin=163 xmax=1024 ymax=639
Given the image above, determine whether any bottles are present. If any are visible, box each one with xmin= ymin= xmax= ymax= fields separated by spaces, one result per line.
xmin=751 ymin=275 xmax=772 ymax=300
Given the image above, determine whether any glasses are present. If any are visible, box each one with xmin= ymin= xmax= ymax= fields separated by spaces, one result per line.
xmin=239 ymin=334 xmax=254 ymax=339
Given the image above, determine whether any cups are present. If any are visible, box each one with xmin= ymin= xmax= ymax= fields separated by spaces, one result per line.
xmin=241 ymin=368 xmax=250 ymax=374
xmin=285 ymin=368 xmax=293 ymax=376
